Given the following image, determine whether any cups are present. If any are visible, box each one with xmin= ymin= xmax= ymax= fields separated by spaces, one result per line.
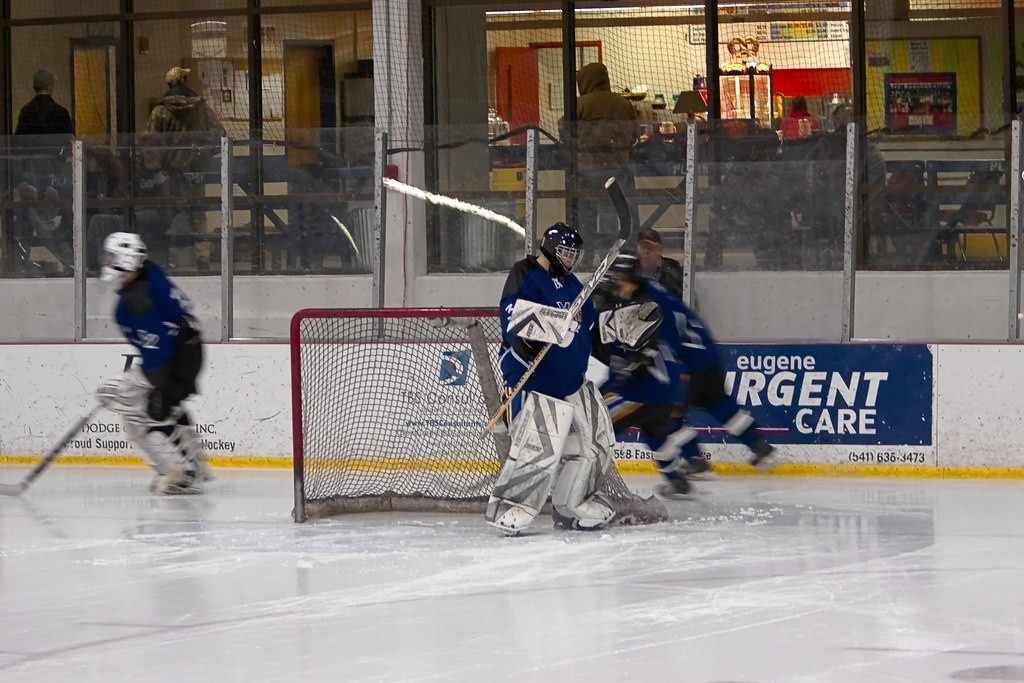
xmin=662 ymin=122 xmax=676 ymax=142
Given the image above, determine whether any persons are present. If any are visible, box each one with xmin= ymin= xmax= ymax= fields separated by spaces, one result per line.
xmin=94 ymin=232 xmax=211 ymax=494
xmin=11 ymin=62 xmax=887 ymax=268
xmin=482 ymin=222 xmax=777 ymax=536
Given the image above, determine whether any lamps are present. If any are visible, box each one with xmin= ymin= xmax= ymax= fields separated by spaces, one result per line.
xmin=673 ymin=91 xmax=707 ymax=123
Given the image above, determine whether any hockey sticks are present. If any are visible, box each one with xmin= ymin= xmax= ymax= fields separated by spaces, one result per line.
xmin=480 ymin=176 xmax=634 ymax=444
xmin=0 ymin=394 xmax=108 ymax=499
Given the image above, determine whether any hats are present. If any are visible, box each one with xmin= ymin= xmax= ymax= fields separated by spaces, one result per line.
xmin=637 ymin=228 xmax=661 ymax=246
xmin=33 ymin=69 xmax=59 ymax=88
xmin=166 ymin=67 xmax=192 ymax=83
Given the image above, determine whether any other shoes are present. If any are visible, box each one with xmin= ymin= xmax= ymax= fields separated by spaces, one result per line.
xmin=751 ymin=441 xmax=780 ymax=468
xmin=198 ymin=256 xmax=209 ymax=270
xmin=680 ymin=457 xmax=718 ymax=479
xmin=654 ymin=479 xmax=695 ymax=499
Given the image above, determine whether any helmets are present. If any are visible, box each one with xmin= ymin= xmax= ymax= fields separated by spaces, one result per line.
xmin=609 ymin=249 xmax=641 ymax=272
xmin=104 ymin=232 xmax=147 ymax=271
xmin=539 ymin=221 xmax=584 ymax=276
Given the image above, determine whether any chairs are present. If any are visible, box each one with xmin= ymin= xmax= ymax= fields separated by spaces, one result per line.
xmin=943 ymin=170 xmax=1005 ymax=261
